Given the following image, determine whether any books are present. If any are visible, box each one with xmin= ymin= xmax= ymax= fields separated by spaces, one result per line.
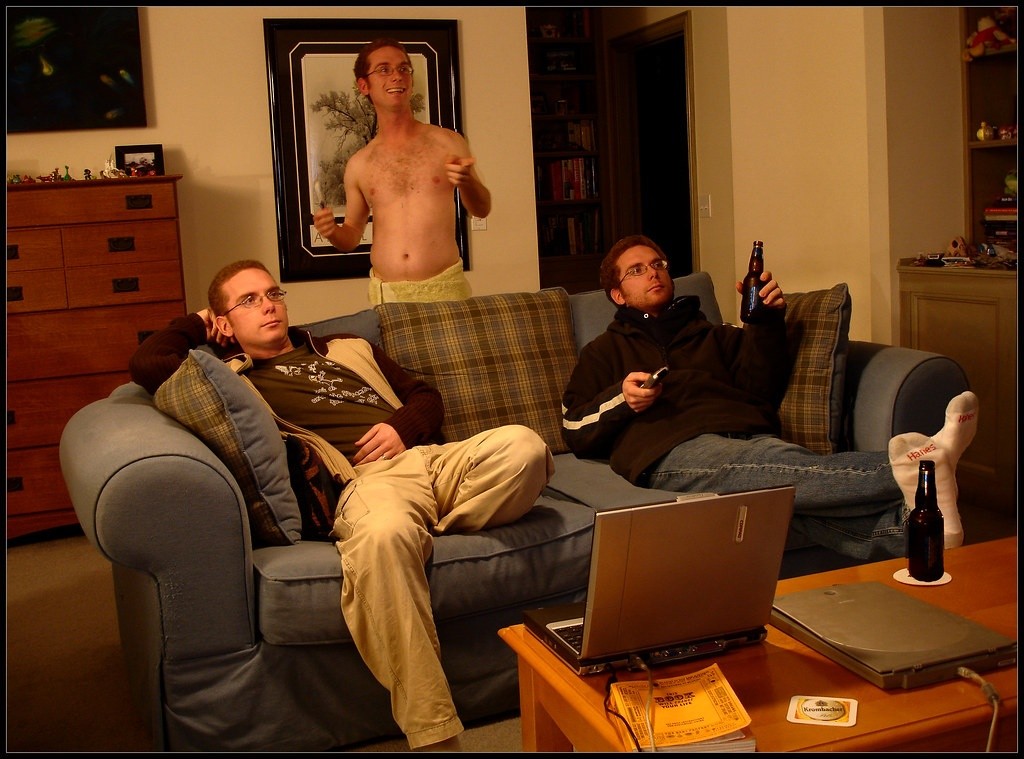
xmin=535 ymin=119 xmax=603 ymax=256
xmin=984 ymin=207 xmax=1018 ymax=216
xmin=610 ymin=663 xmax=756 ymax=753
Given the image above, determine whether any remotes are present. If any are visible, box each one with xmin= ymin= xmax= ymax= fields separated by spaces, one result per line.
xmin=640 ymin=367 xmax=669 ymax=390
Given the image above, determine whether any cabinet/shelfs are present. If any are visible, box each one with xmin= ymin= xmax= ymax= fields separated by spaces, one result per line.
xmin=7 ymin=171 xmax=186 ymax=551
xmin=526 ymin=10 xmax=617 ymax=292
xmin=897 ymin=9 xmax=1017 ymax=524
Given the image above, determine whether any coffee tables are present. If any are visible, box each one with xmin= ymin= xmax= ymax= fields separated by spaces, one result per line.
xmin=498 ymin=537 xmax=1017 ymax=753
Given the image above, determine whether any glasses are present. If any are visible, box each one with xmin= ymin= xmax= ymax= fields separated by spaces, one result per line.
xmin=223 ymin=289 xmax=288 ymax=316
xmin=615 ymin=259 xmax=669 ymax=292
xmin=363 ymin=64 xmax=414 ymax=79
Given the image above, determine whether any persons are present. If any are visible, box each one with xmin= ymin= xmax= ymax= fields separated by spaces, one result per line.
xmin=130 ymin=261 xmax=557 ymax=753
xmin=311 ymin=39 xmax=492 ymax=304
xmin=562 ymin=235 xmax=979 ymax=561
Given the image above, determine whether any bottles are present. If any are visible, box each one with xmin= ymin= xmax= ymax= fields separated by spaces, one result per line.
xmin=740 ymin=241 xmax=767 ymax=324
xmin=909 ymin=460 xmax=944 ymax=582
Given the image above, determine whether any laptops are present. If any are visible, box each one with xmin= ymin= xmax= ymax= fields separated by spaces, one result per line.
xmin=523 ymin=484 xmax=798 ymax=675
xmin=769 ymin=582 xmax=1017 ymax=689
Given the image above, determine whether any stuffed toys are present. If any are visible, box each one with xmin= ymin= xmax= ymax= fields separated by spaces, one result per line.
xmin=964 ymin=17 xmax=1015 ymax=60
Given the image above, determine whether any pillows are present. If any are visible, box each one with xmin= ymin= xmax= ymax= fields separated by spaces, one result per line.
xmin=752 ymin=283 xmax=855 ymax=454
xmin=154 ymin=346 xmax=300 ymax=553
xmin=379 ymin=283 xmax=584 ymax=454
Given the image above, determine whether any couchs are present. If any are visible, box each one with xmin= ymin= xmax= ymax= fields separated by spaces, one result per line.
xmin=56 ymin=293 xmax=977 ymax=752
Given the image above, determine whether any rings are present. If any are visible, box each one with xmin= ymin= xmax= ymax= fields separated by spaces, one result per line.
xmin=382 ymin=454 xmax=388 ymax=460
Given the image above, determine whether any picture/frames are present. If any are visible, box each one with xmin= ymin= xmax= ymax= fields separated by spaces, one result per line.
xmin=260 ymin=17 xmax=474 ymax=285
xmin=114 ymin=144 xmax=167 ymax=176
